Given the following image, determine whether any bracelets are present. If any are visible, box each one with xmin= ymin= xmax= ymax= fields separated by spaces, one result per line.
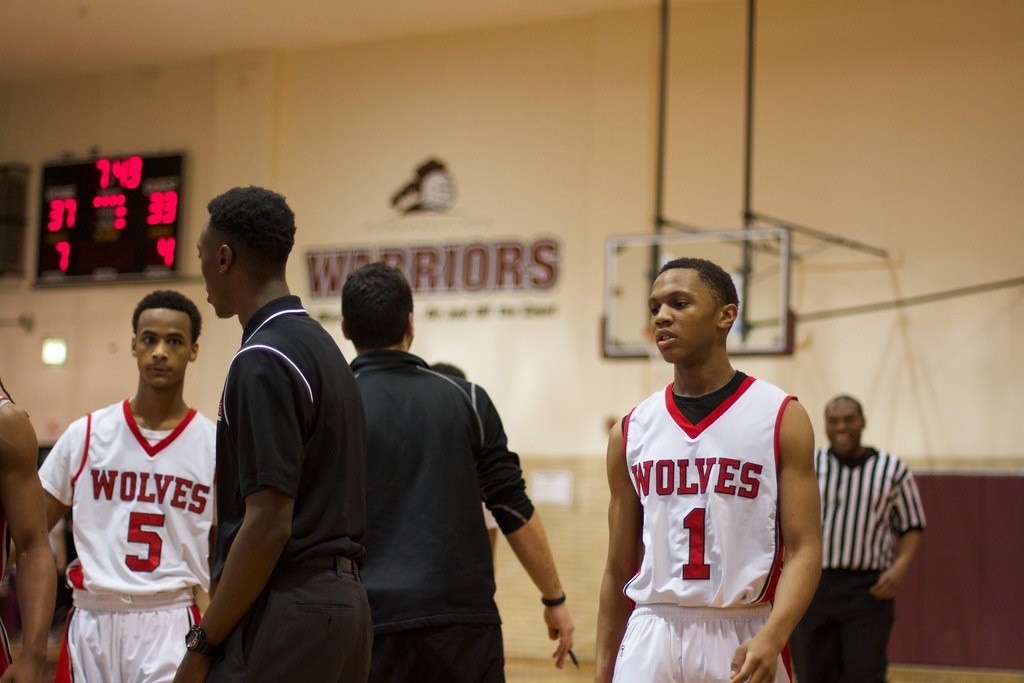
xmin=541 ymin=592 xmax=565 ymax=606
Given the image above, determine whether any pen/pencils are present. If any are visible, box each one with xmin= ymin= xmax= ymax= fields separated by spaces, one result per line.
xmin=569 ymin=650 xmax=579 ymax=669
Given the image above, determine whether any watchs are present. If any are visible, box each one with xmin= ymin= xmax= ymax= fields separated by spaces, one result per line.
xmin=184 ymin=625 xmax=219 ymax=660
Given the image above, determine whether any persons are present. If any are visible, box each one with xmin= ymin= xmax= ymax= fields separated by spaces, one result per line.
xmin=0 ymin=379 xmax=57 ymax=683
xmin=172 ymin=186 xmax=370 ymax=683
xmin=48 ymin=518 xmax=65 ymax=643
xmin=338 ymin=262 xmax=576 ymax=683
xmin=790 ymin=397 xmax=925 ymax=683
xmin=6 ymin=291 xmax=216 ymax=683
xmin=593 ymin=258 xmax=821 ymax=682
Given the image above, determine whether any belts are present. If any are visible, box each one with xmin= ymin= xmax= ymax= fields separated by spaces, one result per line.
xmin=300 ymin=555 xmax=359 ymax=575
